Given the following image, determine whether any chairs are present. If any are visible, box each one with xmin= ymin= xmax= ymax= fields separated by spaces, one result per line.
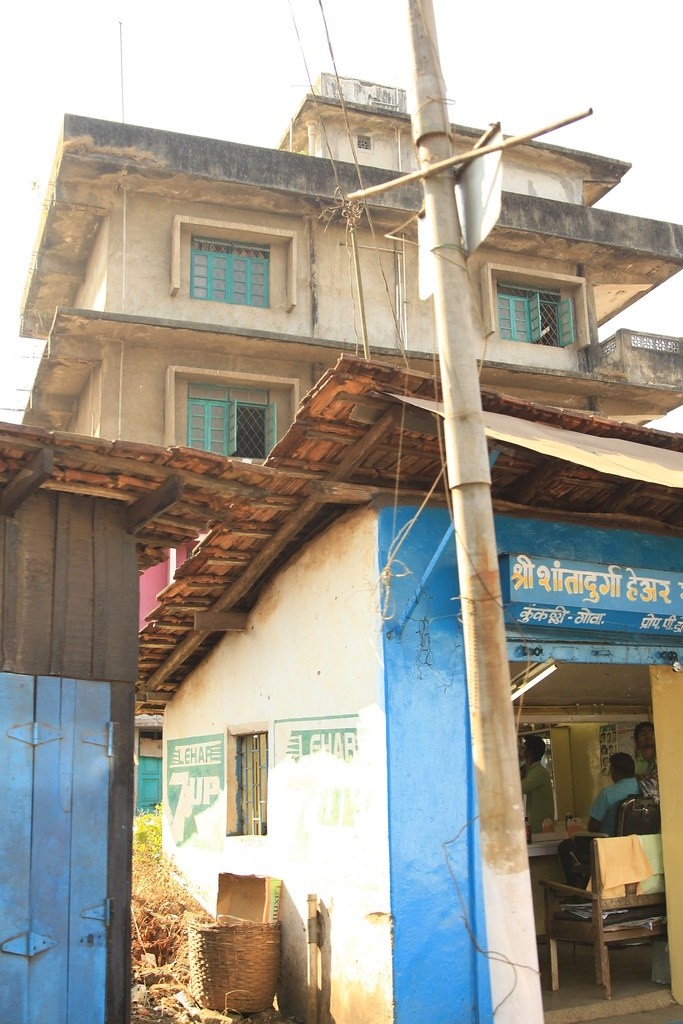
xmin=539 ymin=832 xmax=666 ymax=1000
xmin=567 ymin=794 xmax=663 ymax=884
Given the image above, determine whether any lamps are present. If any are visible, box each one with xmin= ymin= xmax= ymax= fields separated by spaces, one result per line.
xmin=510 ymin=656 xmax=560 ymax=701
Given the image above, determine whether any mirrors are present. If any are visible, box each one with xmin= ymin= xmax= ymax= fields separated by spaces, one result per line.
xmin=515 ymin=721 xmax=655 ymax=840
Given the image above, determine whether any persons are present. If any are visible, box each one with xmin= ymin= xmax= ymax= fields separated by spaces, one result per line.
xmin=518 ymin=735 xmax=552 ymax=833
xmin=632 ymin=721 xmax=657 ymax=777
xmin=599 ymin=730 xmax=618 ymax=774
xmin=587 ymin=753 xmax=643 ymax=837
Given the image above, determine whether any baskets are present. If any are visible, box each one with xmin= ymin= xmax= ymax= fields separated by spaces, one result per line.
xmin=188 ymin=921 xmax=281 ymax=1013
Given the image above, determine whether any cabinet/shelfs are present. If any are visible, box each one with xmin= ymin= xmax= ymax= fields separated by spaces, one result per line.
xmin=519 ymin=726 xmax=576 ymax=824
